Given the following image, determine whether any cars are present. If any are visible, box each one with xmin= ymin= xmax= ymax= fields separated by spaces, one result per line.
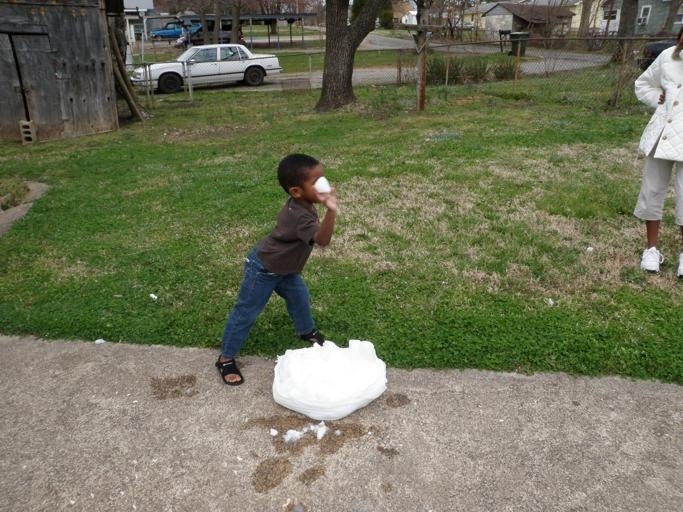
xmin=584 ymin=28 xmax=604 ymax=37
xmin=452 ymin=20 xmax=474 ymax=30
xmin=635 ymin=39 xmax=678 ymax=72
xmin=128 ymin=19 xmax=284 ymax=96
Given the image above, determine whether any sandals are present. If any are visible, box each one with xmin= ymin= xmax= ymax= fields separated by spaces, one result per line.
xmin=304 ymin=331 xmax=326 ymax=345
xmin=215 ymin=354 xmax=241 ymax=385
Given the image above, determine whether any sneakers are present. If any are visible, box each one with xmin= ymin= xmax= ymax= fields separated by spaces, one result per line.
xmin=641 ymin=246 xmax=660 ymax=272
xmin=675 ymin=253 xmax=682 ymax=278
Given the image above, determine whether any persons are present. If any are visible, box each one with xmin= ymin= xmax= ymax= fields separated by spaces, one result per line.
xmin=215 ymin=155 xmax=341 ymax=385
xmin=631 ymin=26 xmax=683 ymax=283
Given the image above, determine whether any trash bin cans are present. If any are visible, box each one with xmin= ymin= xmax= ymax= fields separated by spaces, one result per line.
xmin=510 ymin=31 xmax=530 ymax=57
